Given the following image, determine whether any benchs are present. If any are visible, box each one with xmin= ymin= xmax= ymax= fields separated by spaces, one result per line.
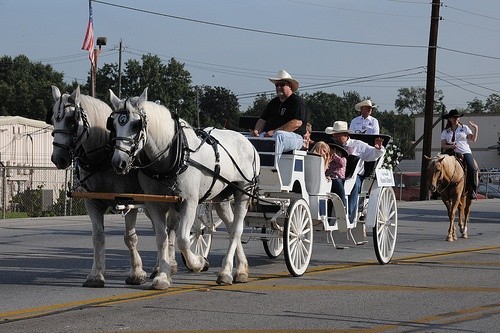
xmin=245 ymin=129 xmax=393 ymax=198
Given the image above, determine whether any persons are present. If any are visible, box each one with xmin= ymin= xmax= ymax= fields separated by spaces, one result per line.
xmin=349 ymin=99 xmax=380 ymax=135
xmin=440 ymin=109 xmax=478 ymax=201
xmin=248 ymin=69 xmax=307 ymax=166
xmin=303 ymin=121 xmax=315 ymax=151
xmin=309 ymin=140 xmax=330 ymax=216
xmin=324 ymin=143 xmax=348 ymax=209
xmin=325 ymin=121 xmax=386 ymax=226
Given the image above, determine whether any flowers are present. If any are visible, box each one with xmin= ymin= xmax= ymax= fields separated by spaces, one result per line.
xmin=378 ymin=142 xmax=405 ymax=170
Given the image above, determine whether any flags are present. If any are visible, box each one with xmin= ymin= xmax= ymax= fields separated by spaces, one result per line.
xmin=81 ymin=0 xmax=95 ymax=71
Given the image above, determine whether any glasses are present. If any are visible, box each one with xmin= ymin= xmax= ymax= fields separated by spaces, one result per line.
xmin=331 ymin=134 xmax=344 ymax=141
xmin=275 ymin=82 xmax=291 ymax=87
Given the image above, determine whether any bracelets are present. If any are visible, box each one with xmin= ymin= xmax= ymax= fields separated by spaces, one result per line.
xmin=273 ymin=129 xmax=277 ymax=133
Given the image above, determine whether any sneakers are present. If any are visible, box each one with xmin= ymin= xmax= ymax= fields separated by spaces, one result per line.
xmin=469 ymin=190 xmax=478 ymax=199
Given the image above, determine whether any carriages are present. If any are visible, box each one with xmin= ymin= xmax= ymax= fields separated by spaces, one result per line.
xmin=48 ymin=83 xmax=398 ymax=289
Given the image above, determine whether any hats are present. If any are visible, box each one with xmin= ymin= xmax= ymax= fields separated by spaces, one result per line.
xmin=325 ymin=120 xmax=353 ymax=134
xmin=447 ymin=109 xmax=465 ymax=117
xmin=269 ymin=69 xmax=299 ymax=91
xmin=355 ymin=99 xmax=376 ymax=110
xmin=327 ymin=141 xmax=349 ymax=157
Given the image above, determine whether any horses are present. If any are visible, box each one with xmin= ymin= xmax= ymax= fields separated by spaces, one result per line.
xmin=424 ymin=153 xmax=477 ymax=242
xmin=51 ymin=85 xmax=261 ymax=290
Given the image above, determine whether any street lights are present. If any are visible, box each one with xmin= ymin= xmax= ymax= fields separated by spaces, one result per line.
xmin=153 ymin=97 xmax=184 ymax=117
